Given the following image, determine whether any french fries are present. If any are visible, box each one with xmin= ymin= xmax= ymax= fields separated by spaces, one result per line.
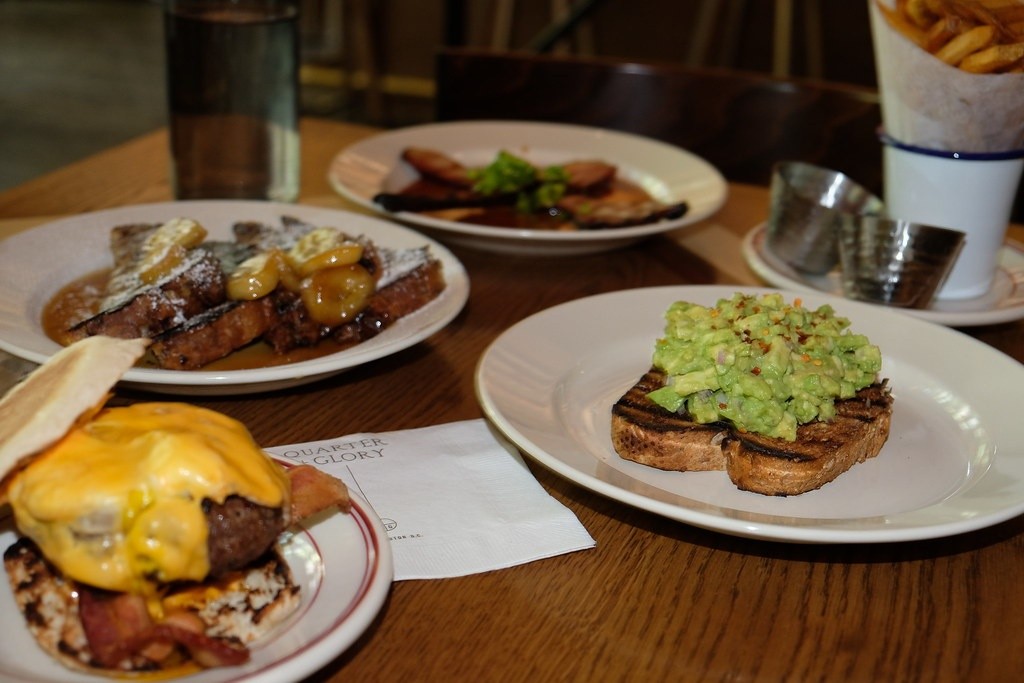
xmin=876 ymin=0 xmax=1024 ymax=77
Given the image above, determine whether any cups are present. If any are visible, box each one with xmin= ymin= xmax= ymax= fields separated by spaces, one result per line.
xmin=766 ymin=160 xmax=883 ymax=277
xmin=164 ymin=0 xmax=301 ymax=201
xmin=880 ymin=133 xmax=1024 ymax=297
xmin=838 ymin=216 xmax=967 ymax=309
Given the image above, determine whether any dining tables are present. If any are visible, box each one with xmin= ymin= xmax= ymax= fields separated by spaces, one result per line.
xmin=0 ymin=114 xmax=1024 ymax=683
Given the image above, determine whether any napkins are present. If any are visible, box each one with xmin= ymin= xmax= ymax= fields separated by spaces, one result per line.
xmin=259 ymin=417 xmax=596 ymax=580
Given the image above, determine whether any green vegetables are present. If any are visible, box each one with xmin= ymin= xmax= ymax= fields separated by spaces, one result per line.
xmin=467 ymin=150 xmax=567 ymax=210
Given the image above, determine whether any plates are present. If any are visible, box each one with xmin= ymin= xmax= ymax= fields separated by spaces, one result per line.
xmin=326 ymin=119 xmax=729 ymax=257
xmin=473 ymin=285 xmax=1024 ymax=545
xmin=0 ymin=200 xmax=471 ymax=394
xmin=0 ymin=449 xmax=395 ymax=683
xmin=741 ymin=220 xmax=1024 ymax=327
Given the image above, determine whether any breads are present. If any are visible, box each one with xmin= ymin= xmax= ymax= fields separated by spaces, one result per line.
xmin=55 ymin=218 xmax=447 ymax=371
xmin=721 ymin=370 xmax=895 ymax=496
xmin=610 ymin=366 xmax=731 ymax=472
